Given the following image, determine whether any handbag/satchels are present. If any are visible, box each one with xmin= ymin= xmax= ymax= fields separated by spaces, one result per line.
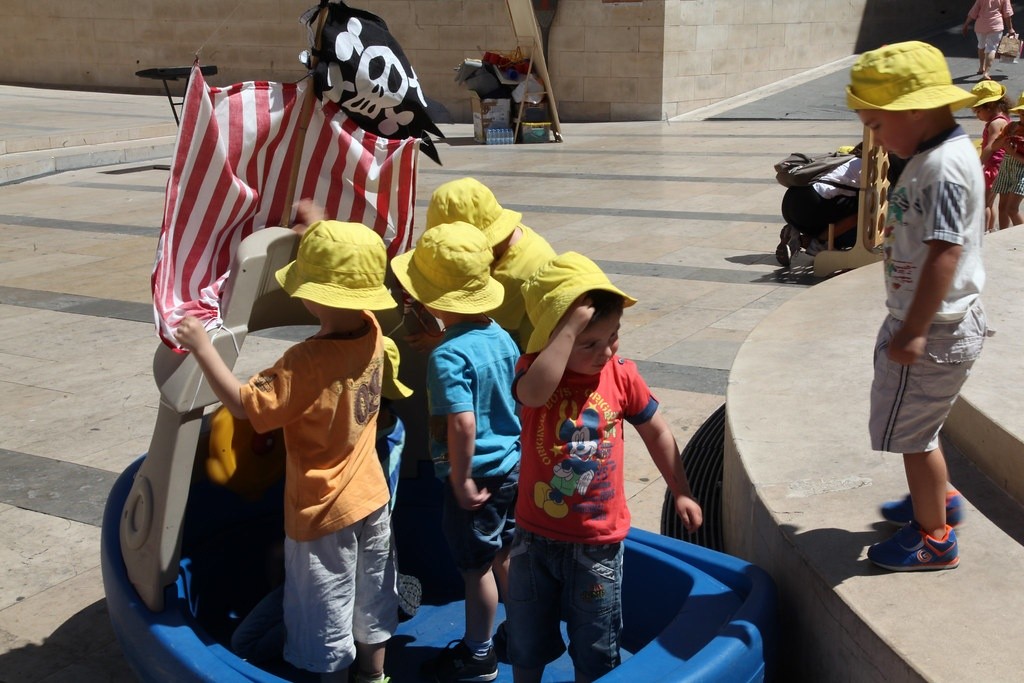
xmin=998 ymin=32 xmax=1020 ymax=58
xmin=999 ymin=33 xmax=1023 ymax=64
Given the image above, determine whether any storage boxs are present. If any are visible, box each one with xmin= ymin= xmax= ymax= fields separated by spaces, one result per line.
xmin=470 ymin=94 xmax=511 ymax=143
xmin=513 ymin=119 xmax=553 ymax=144
xmin=514 ymin=101 xmax=550 ymax=123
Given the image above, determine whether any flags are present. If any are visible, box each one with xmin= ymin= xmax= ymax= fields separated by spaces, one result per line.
xmin=150 ymin=53 xmax=428 ymax=354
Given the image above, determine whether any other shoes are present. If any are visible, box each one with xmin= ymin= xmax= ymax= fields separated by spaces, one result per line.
xmin=353 ymin=672 xmax=392 ymax=683
xmin=983 ymin=72 xmax=991 ymax=80
xmin=979 ymin=68 xmax=985 ymax=73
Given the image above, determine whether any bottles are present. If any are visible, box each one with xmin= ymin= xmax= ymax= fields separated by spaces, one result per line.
xmin=485 ymin=127 xmax=515 ymax=145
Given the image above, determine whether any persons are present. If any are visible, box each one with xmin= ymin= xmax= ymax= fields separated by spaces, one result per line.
xmin=497 ymin=249 xmax=704 ymax=682
xmin=422 ymin=172 xmax=565 ymax=667
xmin=962 ymin=0 xmax=1016 ymax=81
xmin=776 ymin=141 xmax=864 ymax=268
xmin=844 ymin=39 xmax=989 ymax=570
xmin=390 ymin=219 xmax=519 ymax=681
xmin=991 ymin=92 xmax=1024 ymax=230
xmin=967 ymin=80 xmax=1016 ymax=236
xmin=177 ymin=198 xmax=398 ymax=682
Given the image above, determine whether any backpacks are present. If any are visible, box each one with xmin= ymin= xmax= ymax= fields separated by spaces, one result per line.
xmin=773 ymin=150 xmax=861 ymax=193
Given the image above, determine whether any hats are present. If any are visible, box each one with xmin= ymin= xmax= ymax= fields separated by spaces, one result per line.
xmin=426 ymin=176 xmax=523 ymax=248
xmin=390 ymin=220 xmax=505 ymax=314
xmin=379 ymin=336 xmax=414 ymax=401
xmin=846 ymin=40 xmax=977 ymax=115
xmin=970 ymin=81 xmax=1007 ymax=106
xmin=274 ymin=219 xmax=398 ymax=311
xmin=1009 ymin=92 xmax=1024 ymax=114
xmin=520 ymin=251 xmax=638 ymax=354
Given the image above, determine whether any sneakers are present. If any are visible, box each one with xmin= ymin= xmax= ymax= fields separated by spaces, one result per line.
xmin=421 ymin=639 xmax=499 ymax=682
xmin=776 ymin=223 xmax=800 ymax=268
xmin=798 ymin=234 xmax=838 ymax=257
xmin=881 ymin=492 xmax=964 ymax=530
xmin=867 ymin=520 xmax=960 ymax=572
xmin=493 ymin=620 xmax=511 ymax=664
xmin=397 ymin=572 xmax=422 ymax=616
